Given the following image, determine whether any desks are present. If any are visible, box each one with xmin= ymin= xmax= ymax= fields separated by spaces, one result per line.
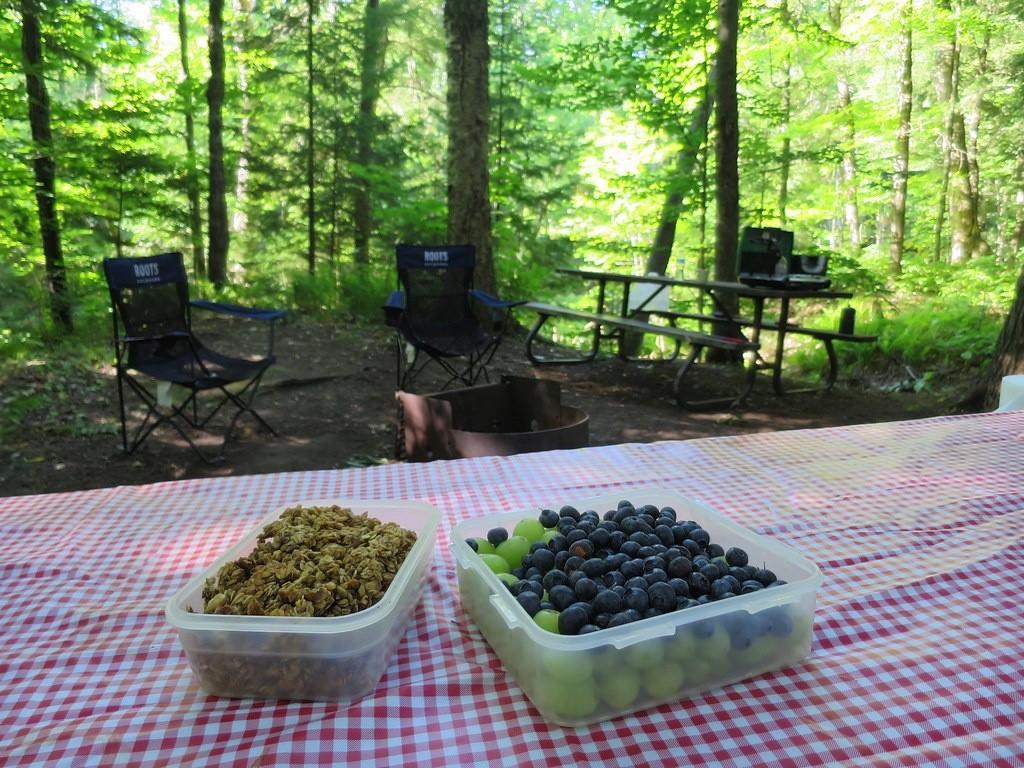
xmin=553 ymin=267 xmax=853 ymax=413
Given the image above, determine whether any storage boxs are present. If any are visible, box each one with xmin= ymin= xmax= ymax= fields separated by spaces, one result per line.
xmin=447 ymin=488 xmax=826 ymax=726
xmin=162 ymin=498 xmax=440 ymax=702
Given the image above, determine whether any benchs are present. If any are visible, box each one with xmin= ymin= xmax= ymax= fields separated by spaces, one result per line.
xmin=632 ymin=310 xmax=879 ymax=397
xmin=522 ymin=301 xmax=762 ymax=412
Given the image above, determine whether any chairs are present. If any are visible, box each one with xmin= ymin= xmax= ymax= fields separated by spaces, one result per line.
xmin=380 ymin=244 xmax=529 ymax=395
xmin=103 ymin=253 xmax=289 ymax=466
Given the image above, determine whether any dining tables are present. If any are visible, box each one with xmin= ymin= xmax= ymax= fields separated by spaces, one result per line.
xmin=0 ymin=407 xmax=1023 ymax=767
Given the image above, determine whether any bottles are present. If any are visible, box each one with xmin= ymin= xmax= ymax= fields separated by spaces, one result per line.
xmin=674 ymin=259 xmax=685 ymax=279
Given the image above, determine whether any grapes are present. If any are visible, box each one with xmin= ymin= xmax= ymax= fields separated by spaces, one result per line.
xmin=465 ymin=501 xmax=811 ymax=716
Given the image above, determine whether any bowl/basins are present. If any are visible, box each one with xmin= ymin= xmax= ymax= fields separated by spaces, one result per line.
xmin=165 ymin=500 xmax=448 ymax=699
xmin=446 ymin=484 xmax=822 ymax=729
